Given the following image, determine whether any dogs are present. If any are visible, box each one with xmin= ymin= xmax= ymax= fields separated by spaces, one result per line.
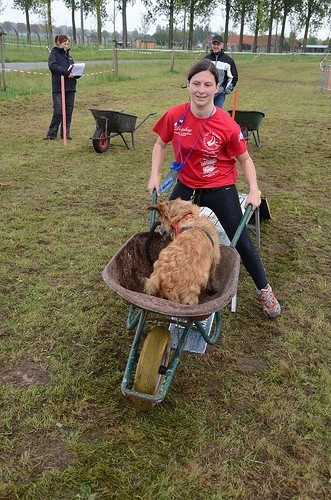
xmin=141 ymin=197 xmax=221 ymax=306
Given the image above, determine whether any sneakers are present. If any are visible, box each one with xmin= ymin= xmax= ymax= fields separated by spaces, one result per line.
xmin=255 ymin=284 xmax=281 ymax=318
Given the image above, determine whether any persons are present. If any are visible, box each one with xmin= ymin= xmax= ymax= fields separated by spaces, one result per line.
xmin=199 ymin=34 xmax=238 ymax=109
xmin=43 ymin=34 xmax=81 ymax=140
xmin=148 ymin=59 xmax=282 ymax=318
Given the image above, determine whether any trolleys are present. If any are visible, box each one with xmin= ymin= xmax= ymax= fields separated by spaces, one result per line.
xmin=228 ymin=109 xmax=266 ymax=149
xmin=102 ymin=190 xmax=262 ymax=412
xmin=87 ymin=106 xmax=157 ymax=154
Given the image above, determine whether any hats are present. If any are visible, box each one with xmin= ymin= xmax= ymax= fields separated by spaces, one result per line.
xmin=211 ymin=35 xmax=223 ymax=43
